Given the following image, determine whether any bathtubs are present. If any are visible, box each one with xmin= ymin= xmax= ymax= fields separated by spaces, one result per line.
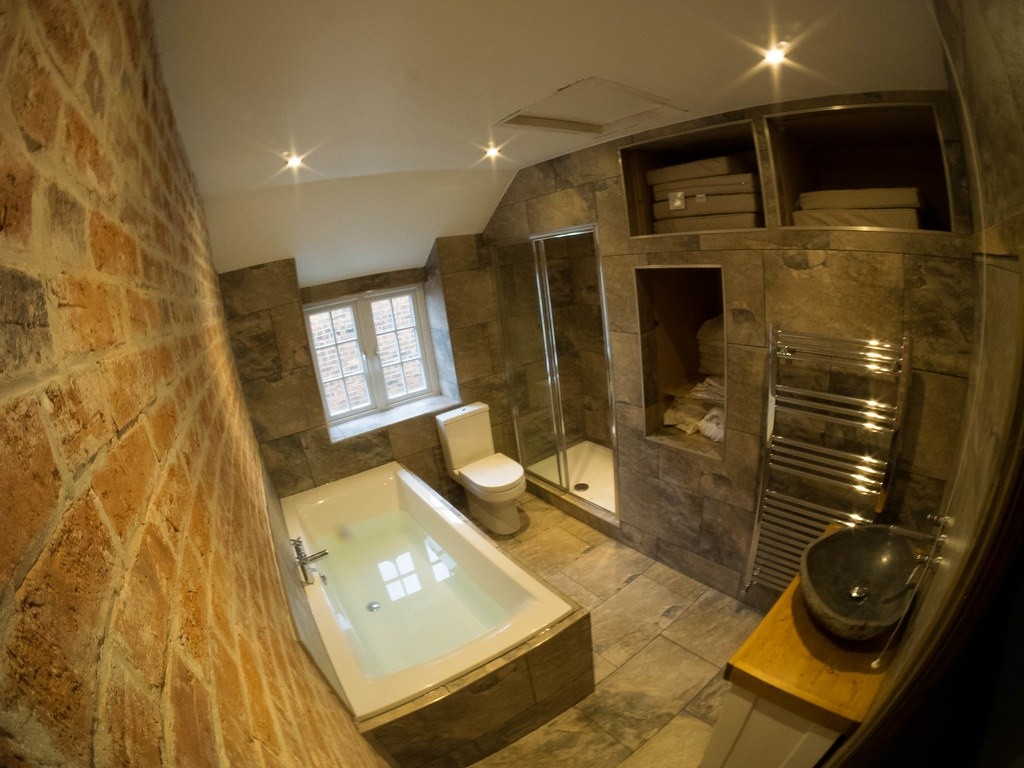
xmin=279 ymin=460 xmax=593 ymax=768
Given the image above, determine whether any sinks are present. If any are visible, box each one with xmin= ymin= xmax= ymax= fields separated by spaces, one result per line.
xmin=801 ymin=524 xmax=925 ymax=641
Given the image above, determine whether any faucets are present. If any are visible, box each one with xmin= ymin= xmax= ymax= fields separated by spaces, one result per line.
xmin=930 ymin=534 xmax=947 ymax=554
xmin=290 ymin=537 xmax=329 ymax=585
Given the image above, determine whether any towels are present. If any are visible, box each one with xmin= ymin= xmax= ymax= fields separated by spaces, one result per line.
xmin=663 ymin=312 xmax=726 ymax=443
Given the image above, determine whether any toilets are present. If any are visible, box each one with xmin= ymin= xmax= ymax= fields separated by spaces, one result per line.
xmin=435 ymin=401 xmax=527 ymax=535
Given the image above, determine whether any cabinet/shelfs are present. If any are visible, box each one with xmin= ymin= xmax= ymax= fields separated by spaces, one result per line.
xmin=617 ymin=90 xmax=974 ymax=237
xmin=697 ymin=684 xmax=843 ymax=768
xmin=633 ymin=264 xmax=726 ymax=461
